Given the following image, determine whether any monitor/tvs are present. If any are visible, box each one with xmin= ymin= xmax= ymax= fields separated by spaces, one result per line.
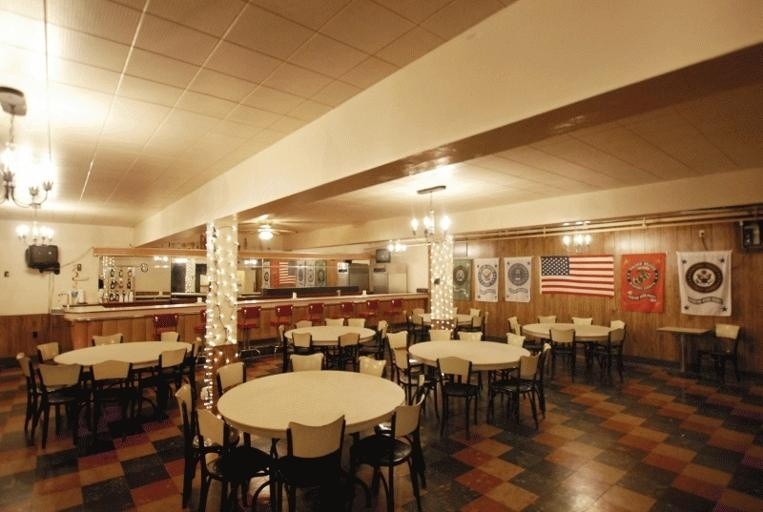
xmin=26 ymin=244 xmax=59 ymax=267
xmin=375 ymin=249 xmax=391 ymax=263
xmin=741 ymin=218 xmax=763 ymax=251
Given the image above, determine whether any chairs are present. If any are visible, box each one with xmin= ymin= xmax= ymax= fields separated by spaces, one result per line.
xmin=694 ymin=323 xmax=741 ymax=387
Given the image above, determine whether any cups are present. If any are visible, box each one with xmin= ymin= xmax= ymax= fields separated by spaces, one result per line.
xmin=293 ymin=292 xmax=297 ymax=299
xmin=118 ymin=291 xmax=134 ymax=302
xmin=362 ymin=289 xmax=367 ymax=295
xmin=197 ymin=297 xmax=202 ymax=303
xmin=336 ymin=290 xmax=341 ymax=296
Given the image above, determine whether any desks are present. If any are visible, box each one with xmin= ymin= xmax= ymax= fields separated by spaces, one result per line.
xmin=656 ymin=325 xmax=712 ymax=380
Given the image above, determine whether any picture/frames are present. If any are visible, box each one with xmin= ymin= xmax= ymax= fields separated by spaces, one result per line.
xmin=735 ymin=221 xmax=762 ymax=253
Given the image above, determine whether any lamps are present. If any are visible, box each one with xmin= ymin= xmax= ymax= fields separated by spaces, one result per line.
xmin=0 ymin=85 xmax=60 ymax=213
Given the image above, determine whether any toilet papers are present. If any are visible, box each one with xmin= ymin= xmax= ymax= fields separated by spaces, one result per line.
xmin=77 ymin=290 xmax=85 ymax=303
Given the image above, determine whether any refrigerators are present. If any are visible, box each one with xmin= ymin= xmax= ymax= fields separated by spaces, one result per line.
xmin=373 ymin=262 xmax=407 ymax=293
